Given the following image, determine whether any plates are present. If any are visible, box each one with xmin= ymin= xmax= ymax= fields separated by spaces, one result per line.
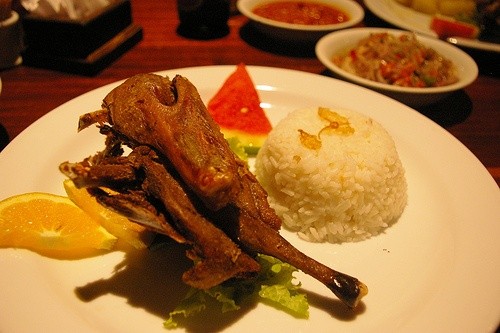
xmin=0 ymin=64 xmax=500 ymax=333
xmin=315 ymin=28 xmax=479 ymax=106
xmin=363 ymin=0 xmax=500 ymax=53
xmin=236 ymin=0 xmax=365 ymax=38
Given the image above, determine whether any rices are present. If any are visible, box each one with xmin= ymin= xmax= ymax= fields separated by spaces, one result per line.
xmin=253 ymin=104 xmax=408 ymax=244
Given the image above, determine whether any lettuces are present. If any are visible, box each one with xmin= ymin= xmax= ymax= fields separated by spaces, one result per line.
xmin=161 ymin=137 xmax=309 ymax=330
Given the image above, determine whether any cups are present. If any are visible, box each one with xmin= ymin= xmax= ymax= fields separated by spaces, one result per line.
xmin=177 ymin=0 xmax=231 ymax=30
xmin=0 ymin=11 xmax=21 ymax=68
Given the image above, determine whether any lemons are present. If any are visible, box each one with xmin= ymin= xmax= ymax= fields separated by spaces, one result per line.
xmin=0 ymin=180 xmax=149 ymax=255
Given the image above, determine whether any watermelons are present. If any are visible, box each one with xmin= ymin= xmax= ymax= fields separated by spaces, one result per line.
xmin=205 ymin=62 xmax=272 ymax=155
